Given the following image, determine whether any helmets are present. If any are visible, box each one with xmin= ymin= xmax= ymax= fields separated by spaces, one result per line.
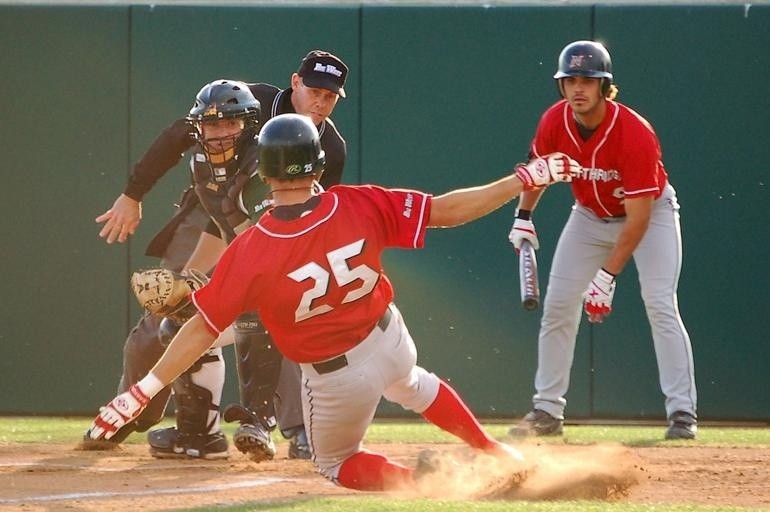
xmin=551 ymin=38 xmax=616 ymax=103
xmin=187 ymin=78 xmax=260 ymax=145
xmin=256 ymin=111 xmax=327 ymax=180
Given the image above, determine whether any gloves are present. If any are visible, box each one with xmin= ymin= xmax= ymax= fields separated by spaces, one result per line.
xmin=513 ymin=150 xmax=584 ymax=194
xmin=506 ymin=206 xmax=543 ymax=258
xmin=83 ymin=382 xmax=151 ymax=444
xmin=580 ymin=266 xmax=619 ymax=326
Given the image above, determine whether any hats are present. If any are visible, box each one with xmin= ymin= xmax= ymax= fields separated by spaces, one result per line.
xmin=295 ymin=48 xmax=349 ymax=100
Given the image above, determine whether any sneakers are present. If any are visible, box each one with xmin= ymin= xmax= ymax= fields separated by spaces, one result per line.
xmin=145 ymin=426 xmax=232 ymax=463
xmin=490 ymin=445 xmax=526 ymax=471
xmin=505 ymin=407 xmax=566 ymax=440
xmin=663 ymin=410 xmax=699 ymax=441
xmin=232 ymin=421 xmax=277 ymax=465
xmin=412 ymin=447 xmax=494 ymax=490
xmin=75 ymin=403 xmax=145 ymax=452
xmin=287 ymin=429 xmax=314 ymax=461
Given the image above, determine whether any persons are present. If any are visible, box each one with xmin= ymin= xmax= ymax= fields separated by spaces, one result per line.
xmin=89 ymin=113 xmax=583 ymax=492
xmin=505 ymin=42 xmax=700 ymax=440
xmin=146 ymin=78 xmax=283 ymax=463
xmin=96 ymin=51 xmax=348 ymax=462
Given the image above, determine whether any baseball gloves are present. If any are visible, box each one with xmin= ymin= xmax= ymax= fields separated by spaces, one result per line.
xmin=132 ymin=270 xmax=213 ymax=324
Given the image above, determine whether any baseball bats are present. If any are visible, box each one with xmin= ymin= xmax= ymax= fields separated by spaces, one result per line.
xmin=519 ymin=237 xmax=541 ymax=309
